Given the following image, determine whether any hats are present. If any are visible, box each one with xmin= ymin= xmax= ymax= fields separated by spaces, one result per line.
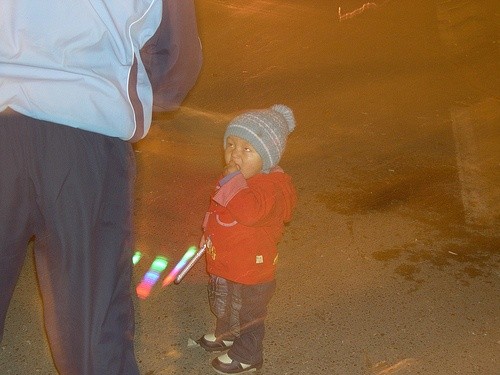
xmin=224 ymin=105 xmax=296 ymax=172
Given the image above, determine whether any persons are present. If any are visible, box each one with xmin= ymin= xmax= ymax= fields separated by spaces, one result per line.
xmin=0 ymin=0 xmax=204 ymax=375
xmin=198 ymin=103 xmax=299 ymax=375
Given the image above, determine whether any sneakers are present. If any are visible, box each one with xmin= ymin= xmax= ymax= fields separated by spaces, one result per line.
xmin=211 ymin=353 xmax=263 ymax=375
xmin=199 ymin=333 xmax=233 ymax=351
